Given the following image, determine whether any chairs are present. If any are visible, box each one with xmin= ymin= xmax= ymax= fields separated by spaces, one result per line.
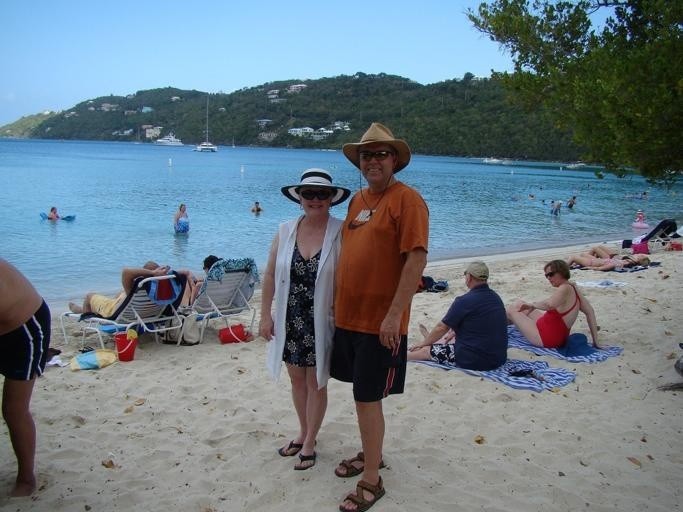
xmin=56 ymin=270 xmax=182 ymax=353
xmin=620 ymin=214 xmax=676 ymax=256
xmin=184 ymin=259 xmax=261 ymax=345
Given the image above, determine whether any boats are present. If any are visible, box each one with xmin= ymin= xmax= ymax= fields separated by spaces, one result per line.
xmin=482 ymin=157 xmax=502 ymax=164
xmin=566 ymin=162 xmax=584 ymax=169
xmin=154 ymin=131 xmax=184 ymax=147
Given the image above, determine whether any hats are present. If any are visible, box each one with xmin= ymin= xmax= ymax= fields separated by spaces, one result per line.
xmin=464 ymin=260 xmax=489 ymax=280
xmin=342 ymin=122 xmax=411 ymax=173
xmin=281 ymin=169 xmax=352 ymax=207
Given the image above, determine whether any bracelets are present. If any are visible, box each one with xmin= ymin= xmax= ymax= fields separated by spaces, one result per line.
xmin=532 ymin=302 xmax=536 ymax=310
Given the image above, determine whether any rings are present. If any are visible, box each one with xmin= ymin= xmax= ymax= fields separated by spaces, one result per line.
xmin=389 ymin=337 xmax=393 ymax=342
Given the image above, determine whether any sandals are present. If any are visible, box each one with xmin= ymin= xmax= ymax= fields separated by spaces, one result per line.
xmin=339 ymin=476 xmax=385 ymax=512
xmin=334 ymin=451 xmax=383 ymax=478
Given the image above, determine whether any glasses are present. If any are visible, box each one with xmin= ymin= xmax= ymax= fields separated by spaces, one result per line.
xmin=358 ymin=150 xmax=393 ymax=161
xmin=544 ymin=271 xmax=556 ymax=278
xmin=299 ymin=189 xmax=333 ymax=200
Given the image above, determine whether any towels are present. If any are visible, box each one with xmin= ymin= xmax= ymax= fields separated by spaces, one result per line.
xmin=506 ymin=324 xmax=624 ymax=365
xmin=414 ymin=358 xmax=577 ymax=393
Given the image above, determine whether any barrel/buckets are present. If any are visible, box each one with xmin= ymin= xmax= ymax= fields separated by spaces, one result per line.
xmin=112 ymin=331 xmax=140 ymax=362
xmin=218 ymin=324 xmax=247 ymax=344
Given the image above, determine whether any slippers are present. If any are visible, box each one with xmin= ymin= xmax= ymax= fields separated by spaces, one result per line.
xmin=294 ymin=449 xmax=316 ymax=470
xmin=78 ymin=345 xmax=94 ymax=353
xmin=279 ymin=439 xmax=316 ymax=456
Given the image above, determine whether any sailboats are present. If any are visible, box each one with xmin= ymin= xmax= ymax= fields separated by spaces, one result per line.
xmin=195 ymin=94 xmax=217 ymax=152
xmin=231 ymin=135 xmax=235 ymax=148
xmin=135 ymin=126 xmax=141 ymax=144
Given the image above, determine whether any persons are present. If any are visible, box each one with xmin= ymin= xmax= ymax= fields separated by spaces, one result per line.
xmin=180 ymin=255 xmax=223 ymax=307
xmin=173 ymin=204 xmax=189 ymax=233
xmin=528 ymin=187 xmax=576 ymax=219
xmin=251 ymin=202 xmax=262 ymax=213
xmin=68 ymin=260 xmax=170 ymax=320
xmin=634 ymin=209 xmax=644 ymax=224
xmin=567 ymin=252 xmax=632 ymax=271
xmin=623 ymin=187 xmax=651 ymax=199
xmin=406 ymin=260 xmax=509 ymax=371
xmin=257 ymin=168 xmax=351 ymax=470
xmin=505 ymin=259 xmax=606 ymax=350
xmin=328 ymin=121 xmax=429 ymax=512
xmin=588 ymin=245 xmax=650 ymax=266
xmin=0 ymin=255 xmax=51 ymax=498
xmin=48 ymin=206 xmax=59 ymax=220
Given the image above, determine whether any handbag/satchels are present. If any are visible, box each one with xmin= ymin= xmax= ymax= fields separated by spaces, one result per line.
xmin=163 ymin=308 xmax=201 ymax=345
